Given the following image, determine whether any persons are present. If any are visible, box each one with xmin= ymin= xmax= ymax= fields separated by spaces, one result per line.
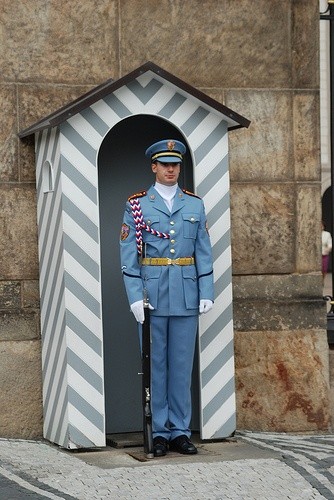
xmin=321 ymin=223 xmax=333 ymax=287
xmin=118 ymin=138 xmax=215 ymax=456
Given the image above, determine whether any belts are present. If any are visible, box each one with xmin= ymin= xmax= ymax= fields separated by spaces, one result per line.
xmin=142 ymin=256 xmax=196 ymax=266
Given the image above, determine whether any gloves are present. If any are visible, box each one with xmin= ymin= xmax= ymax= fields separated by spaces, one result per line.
xmin=199 ymin=297 xmax=215 ymax=314
xmin=130 ymin=298 xmax=155 ymax=325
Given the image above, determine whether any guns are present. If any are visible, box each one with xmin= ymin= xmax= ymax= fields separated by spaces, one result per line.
xmin=137 ymin=242 xmax=154 ymax=459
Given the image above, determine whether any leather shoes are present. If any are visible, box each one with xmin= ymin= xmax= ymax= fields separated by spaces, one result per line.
xmin=153 ymin=436 xmax=170 ymax=457
xmin=170 ymin=434 xmax=198 ymax=455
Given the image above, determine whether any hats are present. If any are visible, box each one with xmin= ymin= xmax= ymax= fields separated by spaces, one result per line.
xmin=144 ymin=140 xmax=188 ymax=165
